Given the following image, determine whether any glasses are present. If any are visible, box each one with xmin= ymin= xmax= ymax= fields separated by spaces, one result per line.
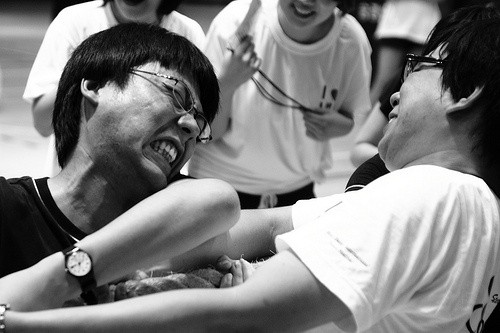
xmin=404 ymin=53 xmax=443 ymax=82
xmin=130 ymin=69 xmax=212 ymax=144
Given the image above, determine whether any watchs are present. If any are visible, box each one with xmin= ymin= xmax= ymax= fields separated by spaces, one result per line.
xmin=60 ymin=244 xmax=97 ymax=297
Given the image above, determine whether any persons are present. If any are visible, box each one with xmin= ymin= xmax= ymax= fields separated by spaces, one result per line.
xmin=0 ymin=22 xmax=240 ymax=312
xmin=0 ymin=0 xmax=500 ymax=333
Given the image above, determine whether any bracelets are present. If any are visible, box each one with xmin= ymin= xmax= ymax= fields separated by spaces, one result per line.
xmin=0 ymin=304 xmax=11 ymax=333
